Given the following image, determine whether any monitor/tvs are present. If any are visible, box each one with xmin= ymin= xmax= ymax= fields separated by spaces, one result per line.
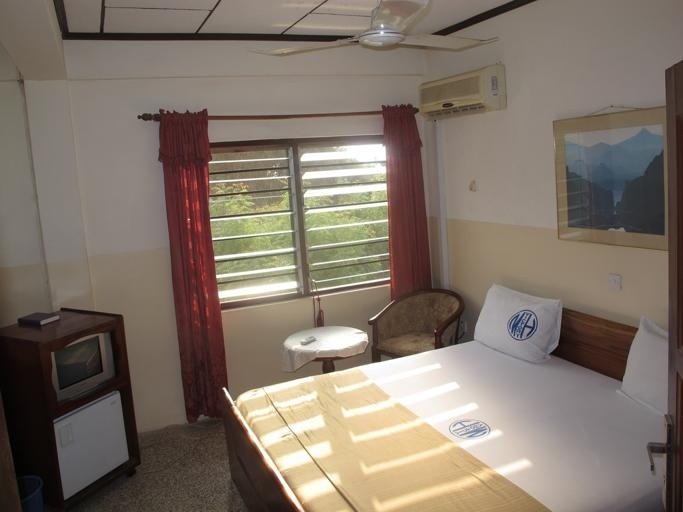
xmin=50 ymin=332 xmax=116 ymax=401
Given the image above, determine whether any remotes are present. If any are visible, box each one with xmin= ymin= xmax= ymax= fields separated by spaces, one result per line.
xmin=300 ymin=336 xmax=315 ymax=344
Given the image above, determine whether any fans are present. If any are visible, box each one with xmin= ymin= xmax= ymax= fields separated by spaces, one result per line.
xmin=269 ymin=0 xmax=499 ymax=58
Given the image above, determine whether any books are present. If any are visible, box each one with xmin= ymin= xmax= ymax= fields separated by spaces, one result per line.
xmin=17 ymin=311 xmax=61 ymax=327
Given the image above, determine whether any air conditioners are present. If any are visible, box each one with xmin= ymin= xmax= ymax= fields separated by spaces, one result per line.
xmin=417 ymin=64 xmax=509 ymax=120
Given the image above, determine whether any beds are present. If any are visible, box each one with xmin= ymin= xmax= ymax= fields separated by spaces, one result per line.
xmin=219 ymin=306 xmax=669 ymax=511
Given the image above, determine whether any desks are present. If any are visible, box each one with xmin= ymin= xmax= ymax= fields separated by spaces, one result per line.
xmin=283 ymin=326 xmax=369 ymax=373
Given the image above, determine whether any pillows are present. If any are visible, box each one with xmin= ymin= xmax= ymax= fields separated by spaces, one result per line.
xmin=616 ymin=316 xmax=670 ymax=418
xmin=473 ymin=282 xmax=564 ymax=362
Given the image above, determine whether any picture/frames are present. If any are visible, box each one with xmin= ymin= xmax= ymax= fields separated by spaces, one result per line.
xmin=551 ymin=106 xmax=668 ymax=249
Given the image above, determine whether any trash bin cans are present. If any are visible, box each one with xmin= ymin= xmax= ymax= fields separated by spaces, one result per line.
xmin=17 ymin=475 xmax=44 ymax=512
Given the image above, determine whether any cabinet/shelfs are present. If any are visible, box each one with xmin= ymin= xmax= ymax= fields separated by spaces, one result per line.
xmin=0 ymin=307 xmax=141 ymax=512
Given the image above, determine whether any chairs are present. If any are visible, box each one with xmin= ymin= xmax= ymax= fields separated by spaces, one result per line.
xmin=368 ymin=288 xmax=465 ymax=362
